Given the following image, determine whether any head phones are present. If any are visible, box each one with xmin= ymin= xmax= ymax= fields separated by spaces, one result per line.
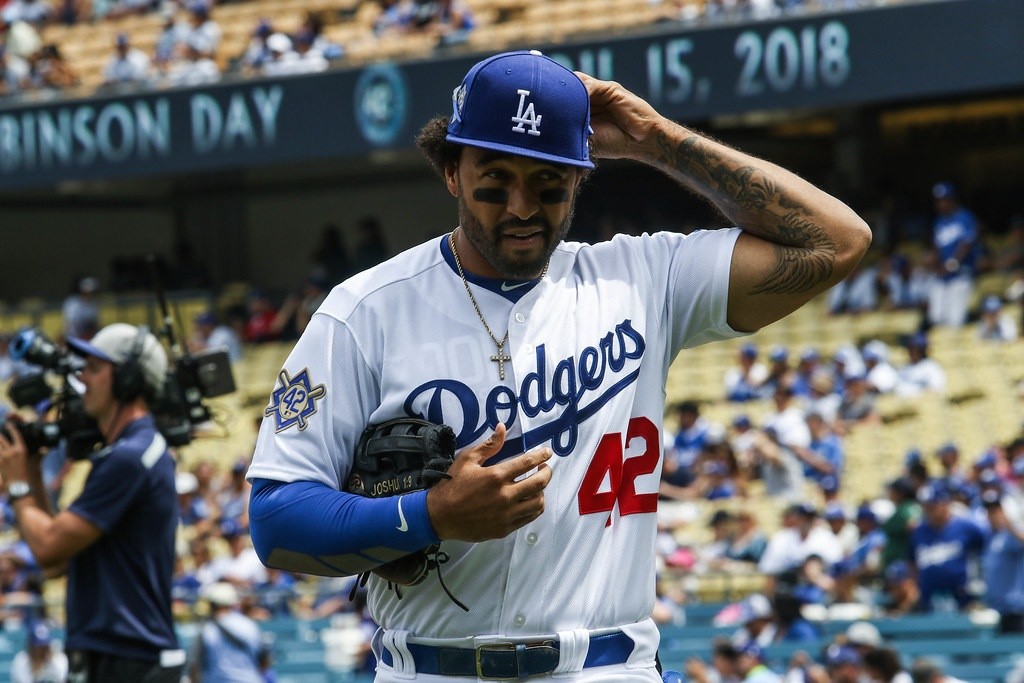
xmin=111 ymin=325 xmax=148 ymax=405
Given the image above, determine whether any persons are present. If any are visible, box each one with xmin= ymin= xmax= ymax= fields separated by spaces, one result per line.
xmin=245 ymin=50 xmax=873 ymax=683
xmin=0 ymin=184 xmax=1024 ymax=683
xmin=0 ymin=0 xmax=476 ymax=98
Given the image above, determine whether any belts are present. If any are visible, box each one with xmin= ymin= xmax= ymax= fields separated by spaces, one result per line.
xmin=371 ymin=619 xmax=660 ymax=681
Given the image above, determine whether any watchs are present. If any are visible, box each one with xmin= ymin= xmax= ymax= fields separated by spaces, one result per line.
xmin=7 ymin=481 xmax=38 ymax=505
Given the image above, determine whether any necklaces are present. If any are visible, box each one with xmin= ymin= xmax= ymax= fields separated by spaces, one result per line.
xmin=450 ymin=226 xmax=550 ymax=381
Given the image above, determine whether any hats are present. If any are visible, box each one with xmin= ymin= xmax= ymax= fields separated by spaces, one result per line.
xmin=671 ymin=180 xmax=1023 ymax=666
xmin=3 ymin=259 xmax=354 ymax=644
xmin=63 ymin=322 xmax=170 ymax=400
xmin=445 ymin=49 xmax=597 ymax=171
